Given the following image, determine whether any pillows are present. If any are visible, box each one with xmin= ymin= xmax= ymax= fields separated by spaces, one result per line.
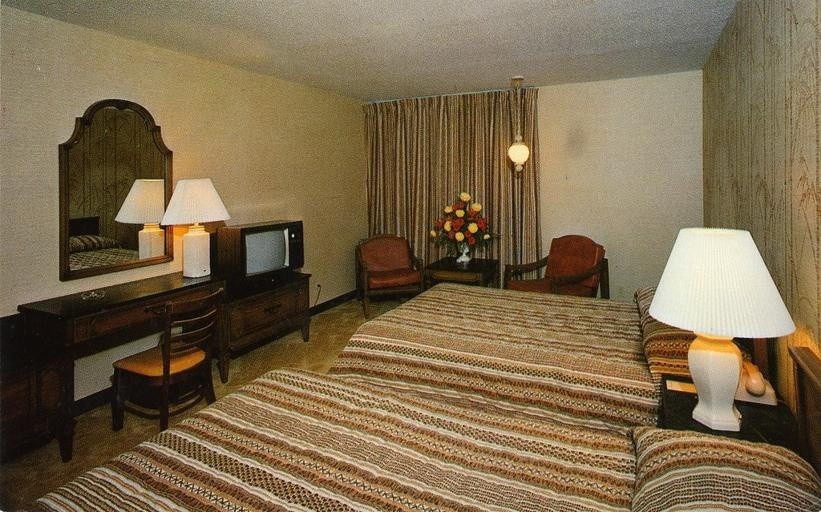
xmin=69 ymin=228 xmax=126 ymax=255
xmin=626 ymin=424 xmax=820 ymax=509
xmin=629 ymin=284 xmax=755 ymax=399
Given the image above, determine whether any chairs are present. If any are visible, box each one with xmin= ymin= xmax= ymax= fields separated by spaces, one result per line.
xmin=109 ymin=286 xmax=226 ymax=438
xmin=353 ymin=231 xmax=430 ymax=319
xmin=502 ymin=230 xmax=612 ymax=298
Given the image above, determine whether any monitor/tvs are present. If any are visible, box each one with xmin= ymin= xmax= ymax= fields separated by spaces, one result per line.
xmin=216 ymin=220 xmax=304 ymax=281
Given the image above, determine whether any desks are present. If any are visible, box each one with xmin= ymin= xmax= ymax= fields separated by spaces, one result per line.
xmin=18 ymin=274 xmax=231 ymax=464
xmin=425 ymin=256 xmax=502 ymax=290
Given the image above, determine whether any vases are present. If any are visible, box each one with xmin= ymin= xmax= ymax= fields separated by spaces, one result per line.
xmin=455 ymin=241 xmax=474 ymax=264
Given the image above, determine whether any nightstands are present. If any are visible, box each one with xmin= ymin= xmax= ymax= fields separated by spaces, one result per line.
xmin=649 ymin=371 xmax=803 ymax=451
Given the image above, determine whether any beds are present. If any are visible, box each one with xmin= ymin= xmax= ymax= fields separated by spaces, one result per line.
xmin=29 ymin=343 xmax=821 ymax=510
xmin=323 ymin=279 xmax=775 ymax=430
xmin=66 ymin=216 xmax=144 ymax=272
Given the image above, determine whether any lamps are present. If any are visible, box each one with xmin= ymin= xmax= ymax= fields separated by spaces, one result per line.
xmin=507 ymin=74 xmax=533 ymax=174
xmin=648 ymin=226 xmax=800 ymax=432
xmin=158 ymin=176 xmax=233 ymax=283
xmin=113 ymin=177 xmax=166 ymax=259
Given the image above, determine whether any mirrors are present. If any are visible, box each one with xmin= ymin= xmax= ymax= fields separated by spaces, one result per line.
xmin=55 ymin=96 xmax=175 ymax=283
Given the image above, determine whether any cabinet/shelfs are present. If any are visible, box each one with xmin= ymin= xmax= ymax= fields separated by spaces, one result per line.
xmin=181 ymin=269 xmax=312 ymax=384
xmin=0 ymin=312 xmax=64 ymax=464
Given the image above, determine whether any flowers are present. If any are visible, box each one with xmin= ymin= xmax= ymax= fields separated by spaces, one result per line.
xmin=429 ymin=190 xmax=506 ymax=261
